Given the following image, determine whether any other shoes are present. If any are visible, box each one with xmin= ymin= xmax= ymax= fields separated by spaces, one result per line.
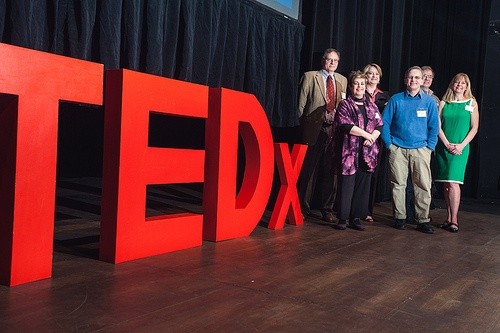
xmin=321 ymin=210 xmax=334 ymax=222
xmin=350 ymin=218 xmax=365 ymax=230
xmin=303 ymin=213 xmax=306 ymax=220
xmin=337 ymin=223 xmax=346 ymax=229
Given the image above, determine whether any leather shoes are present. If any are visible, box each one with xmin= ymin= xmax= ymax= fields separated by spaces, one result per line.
xmin=395 ymin=219 xmax=406 ymax=230
xmin=438 ymin=220 xmax=452 ymax=228
xmin=417 ymin=222 xmax=433 ymax=233
xmin=450 ymin=220 xmax=459 ymax=232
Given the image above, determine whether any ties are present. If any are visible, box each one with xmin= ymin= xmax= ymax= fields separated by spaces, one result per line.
xmin=326 ymin=76 xmax=335 ymax=112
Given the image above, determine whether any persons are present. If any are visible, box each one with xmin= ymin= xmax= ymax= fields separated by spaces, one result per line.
xmin=334 ymin=70 xmax=384 ymax=231
xmin=298 ymin=48 xmax=347 ymax=223
xmin=438 ymin=73 xmax=478 ymax=233
xmin=381 ymin=65 xmax=440 ymax=234
xmin=361 ymin=64 xmax=440 ymax=223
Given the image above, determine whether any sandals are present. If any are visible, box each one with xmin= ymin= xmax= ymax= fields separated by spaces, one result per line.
xmin=366 ymin=216 xmax=373 ymax=222
